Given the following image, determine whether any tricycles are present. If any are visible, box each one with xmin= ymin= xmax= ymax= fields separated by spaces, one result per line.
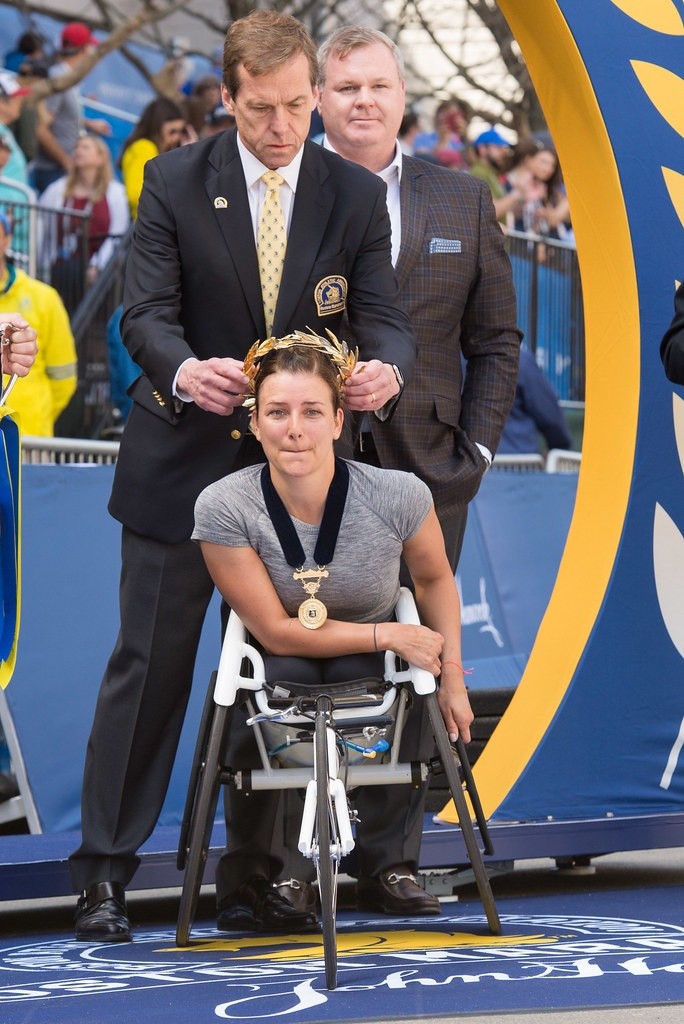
xmin=172 ymin=578 xmax=502 ymax=990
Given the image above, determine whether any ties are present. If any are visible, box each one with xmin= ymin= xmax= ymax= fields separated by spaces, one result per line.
xmin=257 ymin=170 xmax=287 ymax=341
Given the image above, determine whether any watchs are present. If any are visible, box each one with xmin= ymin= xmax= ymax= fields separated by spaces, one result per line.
xmin=393 ymin=365 xmax=405 ymax=389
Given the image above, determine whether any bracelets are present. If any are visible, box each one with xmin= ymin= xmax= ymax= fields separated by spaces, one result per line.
xmin=372 ymin=623 xmax=382 ymax=654
xmin=440 ymin=660 xmax=473 ymax=675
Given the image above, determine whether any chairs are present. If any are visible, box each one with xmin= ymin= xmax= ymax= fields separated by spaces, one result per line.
xmin=172 ymin=585 xmax=505 ymax=990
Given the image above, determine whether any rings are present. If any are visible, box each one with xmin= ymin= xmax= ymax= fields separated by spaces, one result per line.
xmin=371 ymin=394 xmax=374 ymax=401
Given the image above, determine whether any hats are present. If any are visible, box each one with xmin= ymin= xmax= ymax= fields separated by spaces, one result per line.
xmin=62 ymin=23 xmax=102 ymax=46
xmin=475 ymin=129 xmax=510 ymax=146
xmin=0 ymin=72 xmax=32 ymax=97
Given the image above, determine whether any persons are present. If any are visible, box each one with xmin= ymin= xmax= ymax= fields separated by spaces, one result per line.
xmin=0 ymin=22 xmax=571 ymax=424
xmin=66 ymin=12 xmax=416 ymax=945
xmin=314 ymin=27 xmax=525 ymax=625
xmin=191 ymin=329 xmax=474 ymax=924
xmin=0 ymin=204 xmax=78 ymax=440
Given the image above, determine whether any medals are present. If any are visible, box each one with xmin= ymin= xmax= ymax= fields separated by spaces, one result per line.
xmin=293 ymin=565 xmax=328 ymax=630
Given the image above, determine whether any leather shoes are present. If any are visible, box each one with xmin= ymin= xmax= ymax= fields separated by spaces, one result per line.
xmin=275 ymin=879 xmax=316 ymax=912
xmin=217 ymin=884 xmax=322 ymax=934
xmin=76 ymin=882 xmax=134 ymax=941
xmin=357 ymin=865 xmax=442 ymax=915
